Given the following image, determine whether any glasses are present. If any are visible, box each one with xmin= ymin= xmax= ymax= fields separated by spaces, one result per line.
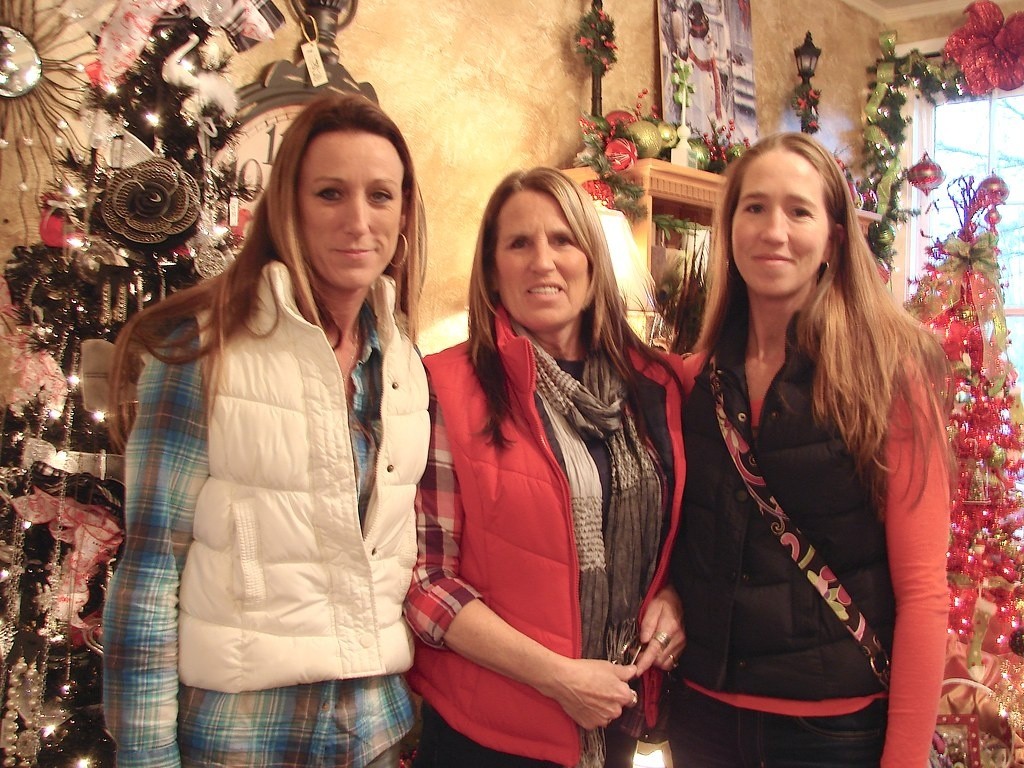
xmin=610 ymin=631 xmax=645 ymax=671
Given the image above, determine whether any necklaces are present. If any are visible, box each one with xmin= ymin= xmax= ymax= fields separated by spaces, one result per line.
xmin=342 ymin=317 xmax=361 ymax=382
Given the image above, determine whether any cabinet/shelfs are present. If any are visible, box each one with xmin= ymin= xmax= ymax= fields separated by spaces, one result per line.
xmin=560 ymin=157 xmax=883 ymax=355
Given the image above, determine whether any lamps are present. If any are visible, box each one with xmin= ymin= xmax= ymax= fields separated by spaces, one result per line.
xmin=794 ymin=29 xmax=821 ymax=82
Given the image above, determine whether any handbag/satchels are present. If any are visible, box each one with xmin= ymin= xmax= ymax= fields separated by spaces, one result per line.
xmin=929 ymin=656 xmax=1023 ymax=768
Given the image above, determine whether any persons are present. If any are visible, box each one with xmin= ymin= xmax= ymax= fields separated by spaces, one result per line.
xmin=688 ymin=133 xmax=948 ymax=768
xmin=105 ymin=95 xmax=431 ymax=768
xmin=413 ymin=167 xmax=687 ymax=768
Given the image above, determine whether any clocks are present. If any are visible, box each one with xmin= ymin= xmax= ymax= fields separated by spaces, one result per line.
xmin=176 ymin=0 xmax=379 ymax=287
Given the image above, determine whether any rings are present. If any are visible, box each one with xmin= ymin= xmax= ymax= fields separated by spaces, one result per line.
xmin=627 ymin=690 xmax=638 ymax=708
xmin=668 ymin=654 xmax=676 ymax=664
xmin=653 ymin=632 xmax=671 ymax=647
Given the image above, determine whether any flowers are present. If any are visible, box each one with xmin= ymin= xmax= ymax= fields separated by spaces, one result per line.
xmin=942 ymin=0 xmax=1024 ymax=96
xmin=575 ymin=4 xmax=619 ymax=73
xmin=99 ymin=156 xmax=201 ymax=252
xmin=791 ymin=84 xmax=823 ymax=134
